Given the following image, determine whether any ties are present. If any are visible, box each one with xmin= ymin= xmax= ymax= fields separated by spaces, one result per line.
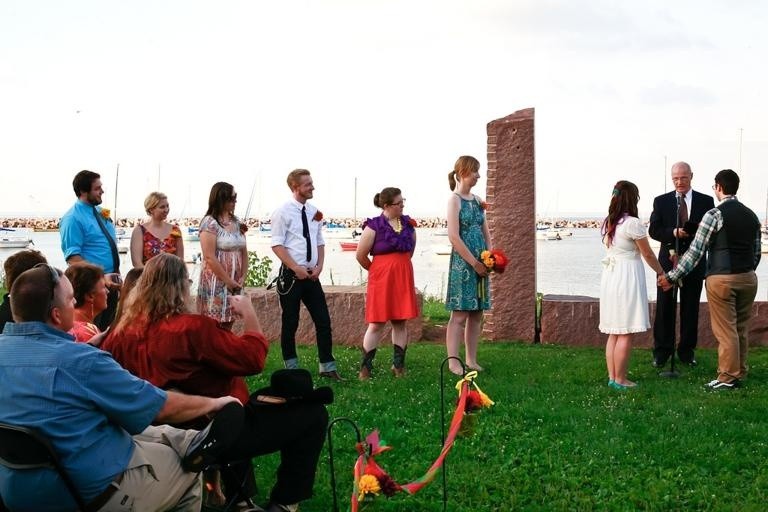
xmin=302 ymin=206 xmax=312 ymax=262
xmin=92 ymin=207 xmax=120 ymax=274
xmin=676 ymin=193 xmax=688 ymax=232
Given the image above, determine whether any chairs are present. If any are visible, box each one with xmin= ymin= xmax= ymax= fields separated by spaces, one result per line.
xmin=0 ymin=420 xmax=92 ymax=511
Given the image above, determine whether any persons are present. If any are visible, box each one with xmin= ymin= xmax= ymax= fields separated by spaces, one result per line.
xmin=2 ymin=264 xmax=243 ymax=512
xmin=62 ymin=171 xmax=124 ymax=330
xmin=444 ymin=156 xmax=489 ymax=376
xmin=598 ymin=182 xmax=667 ymax=389
xmin=199 ymin=182 xmax=248 ymax=335
xmin=58 ymin=264 xmax=107 ymax=347
xmin=272 ymin=170 xmax=346 ymax=384
xmin=129 ymin=192 xmax=184 ymax=269
xmin=0 ymin=250 xmax=46 ymax=331
xmin=647 ymin=161 xmax=714 ymax=370
xmin=100 ymin=253 xmax=332 ymax=512
xmin=657 ymin=171 xmax=763 ymax=391
xmin=357 ymin=187 xmax=418 ymax=379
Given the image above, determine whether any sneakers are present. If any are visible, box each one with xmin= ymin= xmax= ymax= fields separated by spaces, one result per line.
xmin=700 ymin=378 xmax=740 ymax=389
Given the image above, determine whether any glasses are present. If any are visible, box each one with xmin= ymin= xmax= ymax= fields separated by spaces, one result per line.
xmin=31 ymin=262 xmax=61 ymax=304
xmin=391 ymin=199 xmax=404 ymax=205
xmin=672 ymin=176 xmax=691 ymax=180
xmin=227 ymin=193 xmax=237 ymax=202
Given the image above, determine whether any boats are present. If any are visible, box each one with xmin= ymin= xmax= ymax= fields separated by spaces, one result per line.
xmin=114 ymin=229 xmax=128 ymax=253
xmin=0 ymin=227 xmax=35 ymax=248
xmin=327 ymin=222 xmax=345 ymax=232
xmin=339 ymin=241 xmax=359 ymax=252
xmin=535 ymin=223 xmax=573 ymax=242
xmin=181 ymin=226 xmax=200 ymax=241
xmin=259 ymin=224 xmax=273 ymax=237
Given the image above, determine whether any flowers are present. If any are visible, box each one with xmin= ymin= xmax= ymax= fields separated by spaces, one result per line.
xmin=480 ymin=247 xmax=510 ymax=275
xmin=457 ymin=377 xmax=485 ymax=412
xmin=357 ymin=442 xmax=403 ymax=502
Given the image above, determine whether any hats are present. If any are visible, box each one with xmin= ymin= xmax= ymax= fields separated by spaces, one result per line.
xmin=247 ymin=367 xmax=337 ymax=406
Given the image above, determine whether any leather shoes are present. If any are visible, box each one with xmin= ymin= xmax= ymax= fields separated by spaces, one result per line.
xmin=608 ymin=380 xmax=615 ymax=387
xmin=652 ymin=351 xmax=668 ymax=367
xmin=614 ymin=382 xmax=641 ymax=390
xmin=681 ymin=360 xmax=698 ymax=367
xmin=320 ymin=370 xmax=347 ymax=383
xmin=180 ymin=402 xmax=245 ymax=472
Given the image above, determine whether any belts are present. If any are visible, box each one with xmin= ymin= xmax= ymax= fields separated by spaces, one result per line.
xmin=84 ymin=471 xmax=125 ymax=512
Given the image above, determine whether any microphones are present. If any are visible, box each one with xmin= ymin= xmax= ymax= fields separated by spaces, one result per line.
xmin=675 ymin=192 xmax=682 ymax=208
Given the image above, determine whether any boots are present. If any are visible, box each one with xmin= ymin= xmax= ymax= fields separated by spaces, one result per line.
xmin=392 ymin=344 xmax=408 ymax=382
xmin=358 ymin=343 xmax=378 ymax=382
